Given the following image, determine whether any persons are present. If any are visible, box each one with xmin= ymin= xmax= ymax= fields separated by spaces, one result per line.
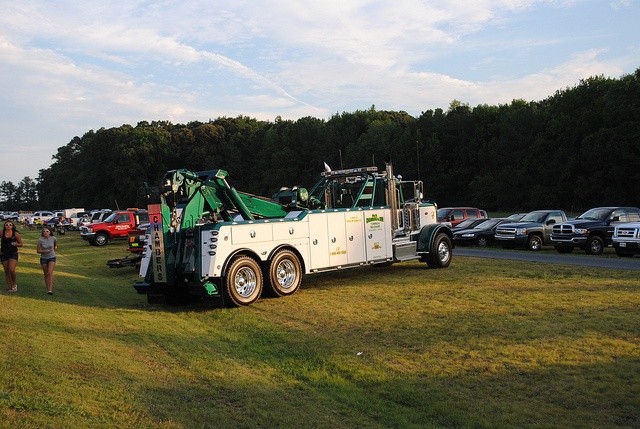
xmin=36 ymin=227 xmax=58 ymax=295
xmin=53 ymin=217 xmax=64 ymax=232
xmin=0 ymin=221 xmax=23 ymax=291
xmin=20 ymin=216 xmax=25 ymax=229
xmin=29 ymin=216 xmax=34 ymax=230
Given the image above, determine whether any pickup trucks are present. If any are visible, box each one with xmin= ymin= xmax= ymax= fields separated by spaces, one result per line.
xmin=495 ymin=210 xmax=567 ymax=251
xmin=79 ymin=211 xmax=150 ymax=246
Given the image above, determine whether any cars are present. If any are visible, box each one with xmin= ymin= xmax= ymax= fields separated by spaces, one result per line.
xmin=453 ymin=220 xmax=519 ymax=248
xmin=509 ymin=214 xmax=527 ymax=221
xmin=450 ymin=220 xmax=489 ymax=231
xmin=31 ymin=210 xmax=55 ymax=221
xmin=0 ymin=210 xmax=32 ymax=223
xmin=50 ymin=208 xmax=111 ymax=229
xmin=611 ymin=222 xmax=640 ymax=257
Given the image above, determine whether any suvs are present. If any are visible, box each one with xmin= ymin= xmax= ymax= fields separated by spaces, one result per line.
xmin=555 ymin=207 xmax=639 ymax=256
xmin=437 ymin=207 xmax=489 ymax=228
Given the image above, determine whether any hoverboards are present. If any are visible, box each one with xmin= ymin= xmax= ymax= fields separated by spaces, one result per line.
xmin=133 ymin=166 xmax=453 ymax=307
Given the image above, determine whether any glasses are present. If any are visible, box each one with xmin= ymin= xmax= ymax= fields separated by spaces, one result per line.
xmin=6 ymin=224 xmax=13 ymax=226
xmin=43 ymin=230 xmax=50 ymax=233
